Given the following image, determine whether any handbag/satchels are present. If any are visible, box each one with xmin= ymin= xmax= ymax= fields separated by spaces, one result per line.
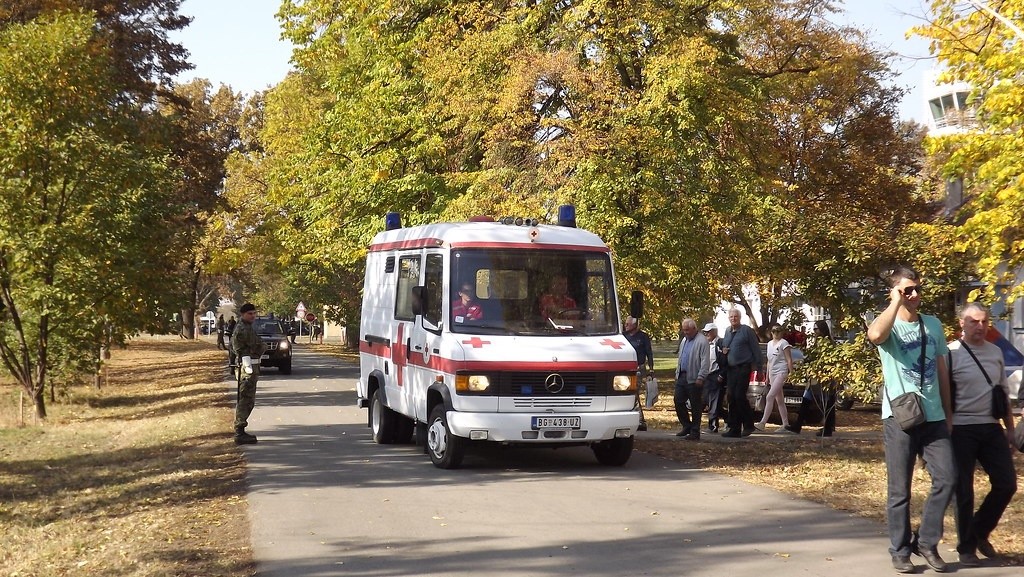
xmin=644 ymin=378 xmax=659 ymax=408
xmin=991 ymin=385 xmax=1009 ymax=419
xmin=889 ymin=392 xmax=927 ymax=430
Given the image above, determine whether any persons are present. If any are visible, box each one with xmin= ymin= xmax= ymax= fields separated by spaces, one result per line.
xmin=867 ymin=268 xmax=958 ymax=574
xmin=673 ymin=318 xmax=711 ymax=440
xmin=943 ymin=301 xmax=1018 ymax=567
xmin=540 ymin=272 xmax=578 ymax=326
xmin=622 ymin=316 xmax=655 ymax=431
xmin=233 ymin=302 xmax=265 ymax=444
xmin=753 ymin=323 xmax=792 ymax=433
xmin=721 ymin=308 xmax=764 ymax=438
xmin=451 ymin=279 xmax=484 ymax=320
xmin=783 ymin=325 xmax=807 ymax=349
xmin=701 ymin=323 xmax=729 ymax=434
xmin=201 ymin=314 xmax=348 ymax=350
xmin=784 ymin=320 xmax=837 ymax=437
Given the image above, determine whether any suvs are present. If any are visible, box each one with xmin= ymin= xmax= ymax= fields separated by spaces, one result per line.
xmin=225 ymin=316 xmax=295 ymax=377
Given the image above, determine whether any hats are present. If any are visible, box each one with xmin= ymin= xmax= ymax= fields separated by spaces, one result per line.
xmin=702 ymin=323 xmax=718 ymax=332
xmin=240 ymin=303 xmax=255 ymax=314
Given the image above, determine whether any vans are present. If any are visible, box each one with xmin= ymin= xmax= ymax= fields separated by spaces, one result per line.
xmin=201 ymin=317 xmax=217 ymax=332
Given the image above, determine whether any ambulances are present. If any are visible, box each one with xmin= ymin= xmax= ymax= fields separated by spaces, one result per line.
xmin=357 ymin=209 xmax=646 ymax=468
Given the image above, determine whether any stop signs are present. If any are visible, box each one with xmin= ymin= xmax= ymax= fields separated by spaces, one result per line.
xmin=306 ymin=313 xmax=314 ymax=322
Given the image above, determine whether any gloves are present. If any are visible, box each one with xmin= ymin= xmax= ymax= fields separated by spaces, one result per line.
xmin=244 ymin=366 xmax=254 ymax=374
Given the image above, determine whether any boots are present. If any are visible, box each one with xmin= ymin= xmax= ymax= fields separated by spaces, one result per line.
xmin=233 ymin=427 xmax=256 ymax=444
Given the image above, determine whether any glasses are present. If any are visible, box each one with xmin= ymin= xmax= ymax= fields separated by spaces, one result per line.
xmin=903 ymin=285 xmax=922 ymax=295
xmin=814 ymin=327 xmax=819 ymax=330
xmin=771 ymin=329 xmax=782 ymax=334
xmin=704 ymin=330 xmax=712 ymax=333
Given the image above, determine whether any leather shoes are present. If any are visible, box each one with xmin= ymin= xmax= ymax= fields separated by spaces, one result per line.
xmin=891 ymin=552 xmax=914 ymax=572
xmin=911 ymin=539 xmax=947 ymax=571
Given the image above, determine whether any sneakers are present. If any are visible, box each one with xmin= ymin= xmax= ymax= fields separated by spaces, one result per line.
xmin=774 ymin=426 xmax=791 ymax=433
xmin=976 ymin=538 xmax=995 ymax=557
xmin=753 ymin=423 xmax=765 ymax=432
xmin=958 ymin=551 xmax=981 ymax=565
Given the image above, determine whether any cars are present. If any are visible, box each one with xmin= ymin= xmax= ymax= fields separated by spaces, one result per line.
xmin=296 ymin=324 xmax=320 ymax=335
xmin=835 ymin=379 xmax=883 ymax=410
xmin=723 ymin=345 xmax=808 ymax=418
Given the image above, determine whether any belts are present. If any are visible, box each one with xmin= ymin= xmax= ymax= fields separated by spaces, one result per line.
xmin=250 ymin=358 xmax=262 ymax=364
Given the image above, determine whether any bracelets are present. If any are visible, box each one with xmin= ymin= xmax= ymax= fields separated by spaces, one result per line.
xmin=649 ymin=370 xmax=654 ymax=373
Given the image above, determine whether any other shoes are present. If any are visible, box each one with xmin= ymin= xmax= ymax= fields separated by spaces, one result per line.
xmin=702 ymin=428 xmax=719 ymax=435
xmin=816 ymin=429 xmax=833 ymax=438
xmin=722 ymin=428 xmax=741 ymax=438
xmin=742 ymin=427 xmax=755 ymax=437
xmin=675 ymin=422 xmax=700 ymax=441
xmin=785 ymin=426 xmax=802 ymax=435
xmin=636 ymin=424 xmax=648 ymax=431
